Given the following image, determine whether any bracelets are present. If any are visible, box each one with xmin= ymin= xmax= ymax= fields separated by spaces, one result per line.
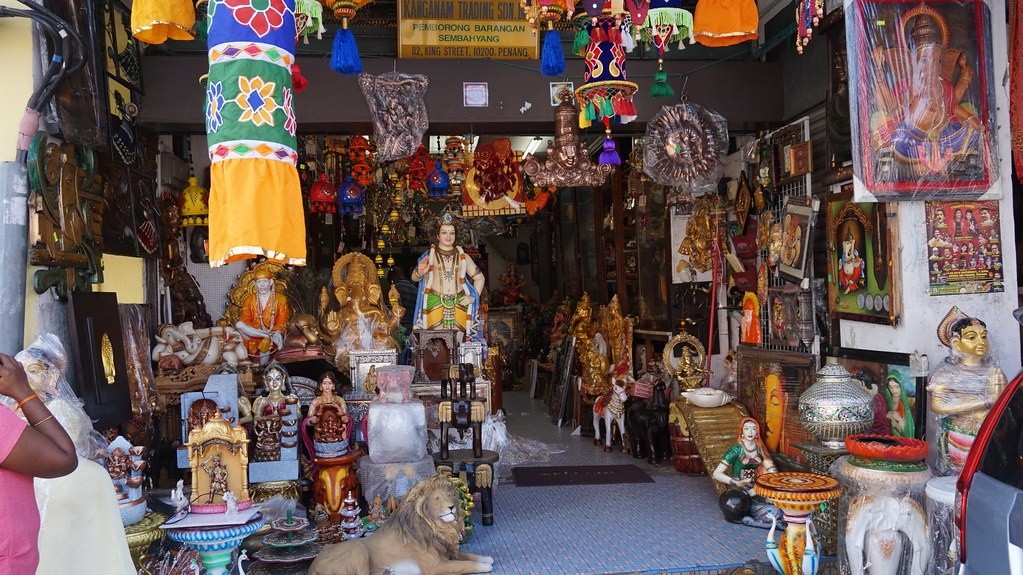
xmin=415 ymin=271 xmax=421 ymax=278
xmin=32 ymin=415 xmax=54 ymax=427
xmin=19 ymin=393 xmax=38 ymax=407
xmin=962 ymin=77 xmax=971 ymax=82
xmin=984 ymin=398 xmax=991 ymax=408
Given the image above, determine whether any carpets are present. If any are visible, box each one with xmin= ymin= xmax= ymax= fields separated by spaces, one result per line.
xmin=510 ymin=464 xmax=655 ymax=487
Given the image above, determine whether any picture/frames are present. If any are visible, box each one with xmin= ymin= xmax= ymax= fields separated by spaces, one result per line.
xmin=820 ymin=342 xmax=928 ymax=443
xmin=733 ymin=170 xmax=754 ymax=235
xmin=550 ymin=335 xmax=576 ymax=427
xmin=789 ymin=140 xmax=814 ymax=177
xmin=823 ymin=191 xmax=904 ymax=330
xmin=774 ymin=196 xmax=820 ymax=289
xmin=736 ymin=345 xmax=817 ymax=470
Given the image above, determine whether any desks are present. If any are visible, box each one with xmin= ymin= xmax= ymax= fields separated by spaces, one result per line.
xmin=430 ymin=396 xmax=499 ymax=526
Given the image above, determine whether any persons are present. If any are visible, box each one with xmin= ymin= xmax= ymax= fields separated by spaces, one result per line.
xmin=308 ymin=374 xmax=349 ymax=442
xmin=202 ymin=456 xmax=228 ymax=503
xmin=712 ymin=418 xmax=777 ymax=497
xmin=551 ymin=295 xmax=628 ymax=391
xmin=236 ymin=266 xmax=289 ymax=364
xmin=0 ymin=353 xmax=78 ymax=575
xmin=928 ymin=305 xmax=1009 ymax=476
xmin=718 ymin=350 xmax=738 ymax=393
xmin=411 ymin=212 xmax=485 ymax=329
xmin=872 ymin=14 xmax=981 ymax=158
xmin=252 ymin=360 xmax=300 ymax=459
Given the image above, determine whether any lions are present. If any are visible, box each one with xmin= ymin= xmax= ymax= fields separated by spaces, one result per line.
xmin=306 ymin=473 xmax=495 ymax=575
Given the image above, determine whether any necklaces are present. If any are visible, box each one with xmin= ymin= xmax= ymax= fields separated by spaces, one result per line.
xmin=435 ymin=247 xmax=459 ymax=308
xmin=741 ymin=441 xmax=757 ymax=452
xmin=256 ymin=290 xmax=275 ymax=331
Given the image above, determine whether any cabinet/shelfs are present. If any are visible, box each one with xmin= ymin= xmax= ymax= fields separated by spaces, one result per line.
xmin=528 ymin=135 xmax=671 ymax=334
